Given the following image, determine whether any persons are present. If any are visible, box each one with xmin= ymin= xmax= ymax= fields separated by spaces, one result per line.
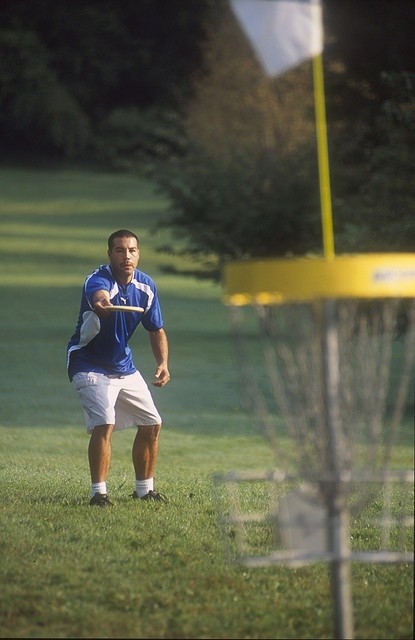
xmin=66 ymin=230 xmax=170 ymax=506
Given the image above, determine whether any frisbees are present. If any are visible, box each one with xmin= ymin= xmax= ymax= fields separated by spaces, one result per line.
xmin=105 ymin=304 xmax=144 ymax=315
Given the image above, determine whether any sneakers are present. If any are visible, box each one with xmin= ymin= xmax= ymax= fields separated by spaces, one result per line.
xmin=131 ymin=490 xmax=168 ymax=505
xmin=89 ymin=493 xmax=113 ymax=507
